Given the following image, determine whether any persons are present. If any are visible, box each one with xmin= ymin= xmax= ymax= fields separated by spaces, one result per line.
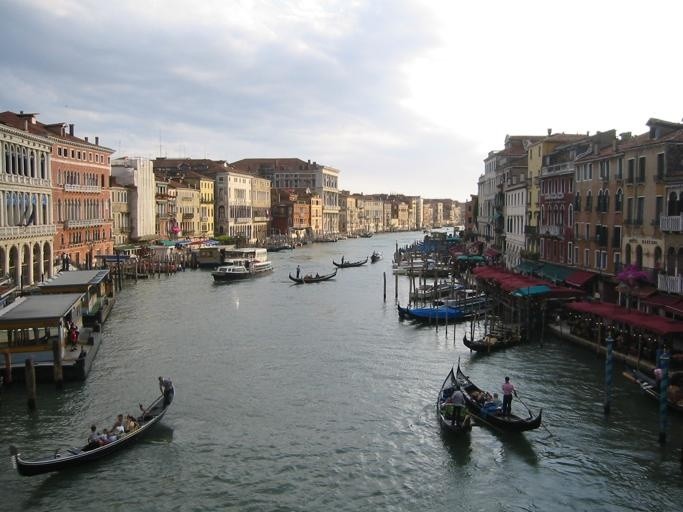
xmin=502 ymin=376 xmax=518 ymax=420
xmin=341 ymin=255 xmax=344 ymax=264
xmin=451 ymin=385 xmax=465 ymax=426
xmin=304 ymin=272 xmax=320 ymax=280
xmin=69 ymin=322 xmax=80 ymax=351
xmin=88 ymin=414 xmax=137 ymax=449
xmin=157 ymin=376 xmax=174 ymax=408
xmin=296 ymin=264 xmax=301 ymax=278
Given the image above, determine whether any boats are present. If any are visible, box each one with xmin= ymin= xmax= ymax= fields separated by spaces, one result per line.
xmin=463 ymin=317 xmax=538 ymax=352
xmin=315 ymin=229 xmax=373 ymax=243
xmin=289 ymin=268 xmax=337 ymax=283
xmin=392 ymin=226 xmax=453 ymax=276
xmin=399 ymin=283 xmax=496 ymax=322
xmin=211 ymin=260 xmax=274 ymax=281
xmin=333 ymin=250 xmax=384 ymax=267
xmin=267 ymin=233 xmax=303 ymax=252
xmin=436 ymin=356 xmax=541 ymax=433
xmin=11 ymin=385 xmax=175 ymax=476
xmin=622 ymin=368 xmax=683 ymax=413
